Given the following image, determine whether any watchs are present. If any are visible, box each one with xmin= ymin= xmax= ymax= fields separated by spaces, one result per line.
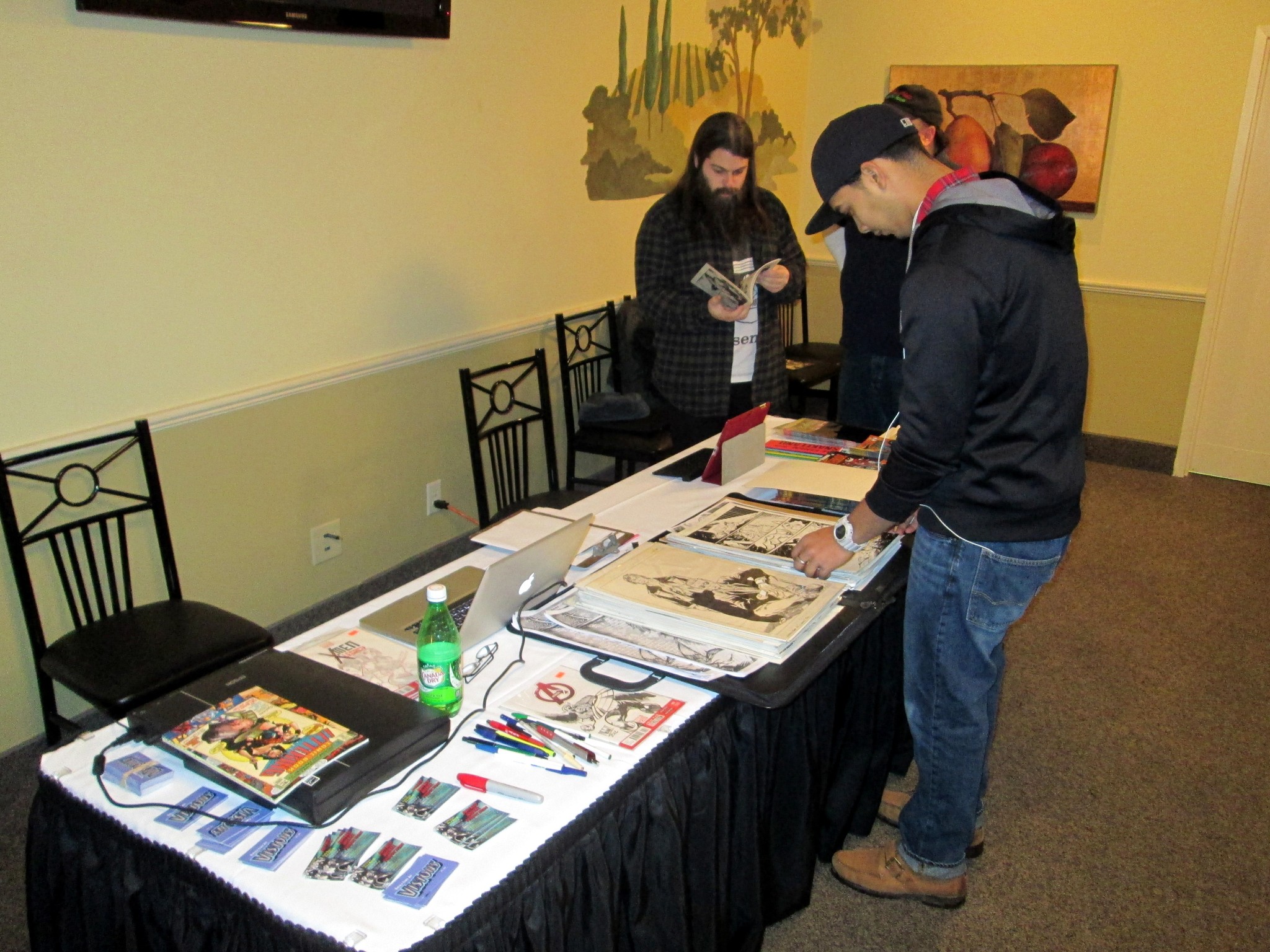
xmin=834 ymin=513 xmax=868 ymax=553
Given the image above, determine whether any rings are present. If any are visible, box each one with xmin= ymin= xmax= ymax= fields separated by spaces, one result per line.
xmin=797 ymin=556 xmax=807 ymax=565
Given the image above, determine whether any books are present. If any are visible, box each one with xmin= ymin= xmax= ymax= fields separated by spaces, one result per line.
xmin=690 ymin=258 xmax=781 ymax=310
xmin=162 ymin=685 xmax=370 ymax=805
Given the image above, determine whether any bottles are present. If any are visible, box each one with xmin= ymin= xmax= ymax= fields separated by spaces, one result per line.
xmin=415 ymin=583 xmax=464 ymax=718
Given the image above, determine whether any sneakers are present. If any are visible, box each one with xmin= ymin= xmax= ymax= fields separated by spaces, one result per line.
xmin=878 ymin=788 xmax=984 ymax=859
xmin=831 ymin=840 xmax=967 ymax=910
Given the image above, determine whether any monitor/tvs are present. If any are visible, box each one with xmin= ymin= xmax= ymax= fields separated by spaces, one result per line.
xmin=74 ymin=0 xmax=451 ymax=39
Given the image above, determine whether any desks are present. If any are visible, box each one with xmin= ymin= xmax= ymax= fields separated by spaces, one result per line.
xmin=24 ymin=415 xmax=916 ymax=952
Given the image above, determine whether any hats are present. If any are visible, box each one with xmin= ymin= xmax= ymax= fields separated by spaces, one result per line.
xmin=885 ymin=84 xmax=952 ymax=142
xmin=805 ymin=103 xmax=917 ymax=235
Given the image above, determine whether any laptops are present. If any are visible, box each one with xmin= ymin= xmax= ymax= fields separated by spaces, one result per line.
xmin=651 ymin=399 xmax=773 ymax=482
xmin=125 ymin=645 xmax=453 ymax=828
xmin=357 ymin=512 xmax=597 ymax=657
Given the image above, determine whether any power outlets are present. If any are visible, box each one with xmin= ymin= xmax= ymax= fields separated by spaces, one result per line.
xmin=426 ymin=479 xmax=441 ymax=515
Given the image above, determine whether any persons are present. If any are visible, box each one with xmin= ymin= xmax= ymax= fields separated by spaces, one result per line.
xmin=635 ymin=111 xmax=807 ymax=462
xmin=792 ymin=103 xmax=1089 ymax=908
xmin=822 ymin=83 xmax=967 ymax=443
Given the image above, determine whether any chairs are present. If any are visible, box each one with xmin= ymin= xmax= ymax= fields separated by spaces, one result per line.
xmin=555 ymin=268 xmax=843 ymax=492
xmin=0 ymin=418 xmax=274 ymax=748
xmin=459 ymin=348 xmax=588 ymax=548
